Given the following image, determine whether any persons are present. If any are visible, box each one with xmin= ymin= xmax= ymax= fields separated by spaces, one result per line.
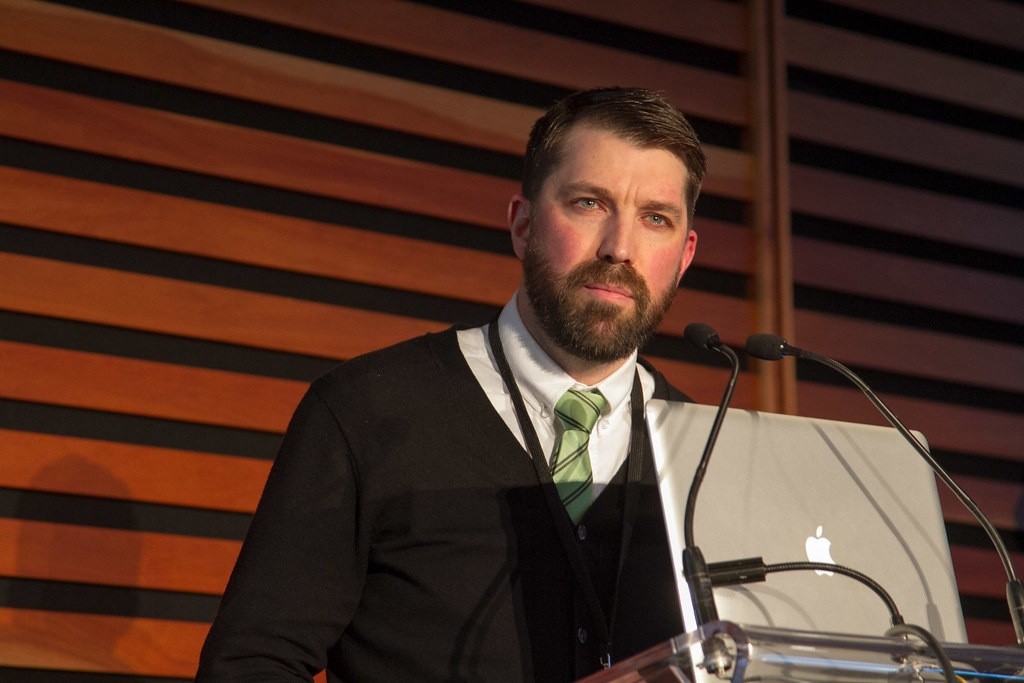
xmin=193 ymin=87 xmax=708 ymax=683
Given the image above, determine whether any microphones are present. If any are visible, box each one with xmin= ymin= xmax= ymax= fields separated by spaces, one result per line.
xmin=744 ymin=334 xmax=1024 ymax=646
xmin=683 ymin=322 xmax=741 ymax=676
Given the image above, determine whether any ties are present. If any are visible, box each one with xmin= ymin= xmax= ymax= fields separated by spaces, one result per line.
xmin=548 ymin=388 xmax=608 ymax=526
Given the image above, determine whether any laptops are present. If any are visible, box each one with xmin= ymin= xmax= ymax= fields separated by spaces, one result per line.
xmin=648 ymin=399 xmax=981 ymax=683
